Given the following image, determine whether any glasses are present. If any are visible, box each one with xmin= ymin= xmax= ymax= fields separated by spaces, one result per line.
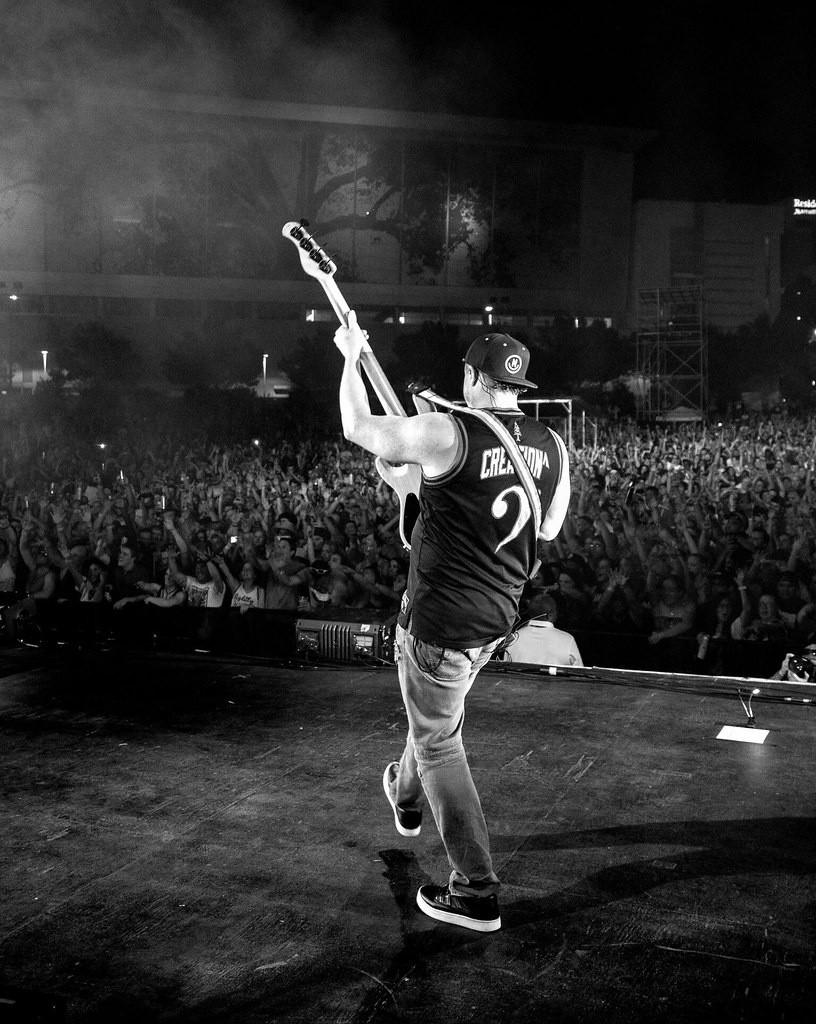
xmin=590 ymin=543 xmax=602 ymax=549
xmin=802 ymin=649 xmax=816 ymax=656
xmin=310 ymin=566 xmax=329 ymax=575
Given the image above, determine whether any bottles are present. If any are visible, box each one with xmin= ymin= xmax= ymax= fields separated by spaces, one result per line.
xmin=696 ymin=636 xmax=709 ymax=660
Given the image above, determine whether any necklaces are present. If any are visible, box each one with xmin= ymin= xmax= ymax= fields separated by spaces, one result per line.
xmin=165 ymin=586 xmax=177 ymax=599
xmin=663 ymin=600 xmax=677 ymax=616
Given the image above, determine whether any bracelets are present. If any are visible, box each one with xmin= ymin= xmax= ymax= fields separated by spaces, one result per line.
xmin=737 ymin=586 xmax=747 ymax=590
xmin=608 ymin=586 xmax=615 ymax=593
xmin=791 ymin=551 xmax=800 ymax=559
xmin=205 ymin=558 xmax=210 ymax=564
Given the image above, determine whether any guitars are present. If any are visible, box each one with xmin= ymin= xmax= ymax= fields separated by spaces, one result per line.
xmin=280 ymin=215 xmax=425 ymax=554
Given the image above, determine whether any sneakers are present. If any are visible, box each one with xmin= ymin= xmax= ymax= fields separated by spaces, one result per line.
xmin=383 ymin=762 xmax=422 ymax=837
xmin=416 ymin=884 xmax=501 ymax=932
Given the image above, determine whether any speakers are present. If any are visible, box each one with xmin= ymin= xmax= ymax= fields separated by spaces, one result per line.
xmin=0 ymin=592 xmax=38 ymax=646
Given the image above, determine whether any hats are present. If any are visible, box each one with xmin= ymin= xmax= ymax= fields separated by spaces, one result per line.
xmin=465 ymin=333 xmax=538 ymax=389
xmin=560 ymin=554 xmax=584 ymax=567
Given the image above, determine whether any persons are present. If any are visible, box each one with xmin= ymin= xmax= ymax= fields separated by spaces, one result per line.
xmin=331 ymin=313 xmax=572 ymax=932
xmin=0 ymin=391 xmax=815 ymax=685
